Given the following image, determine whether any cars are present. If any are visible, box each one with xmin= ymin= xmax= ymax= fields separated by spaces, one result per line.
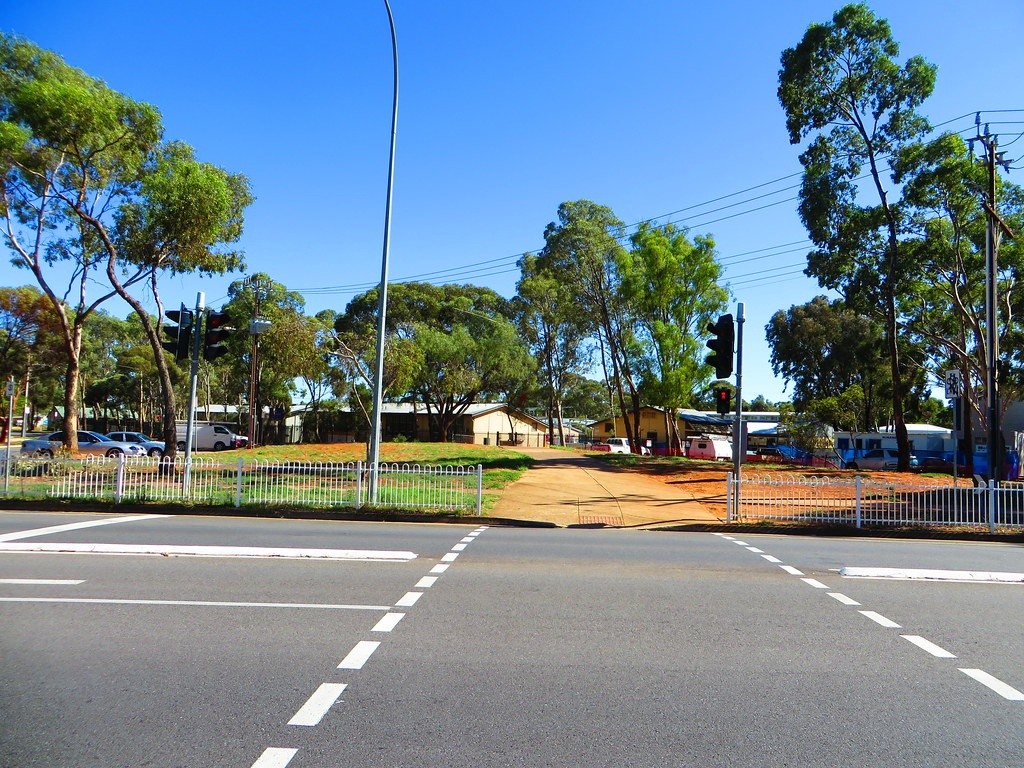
xmin=20 ymin=429 xmax=148 ymax=475
xmin=235 ymin=435 xmax=249 ymax=448
xmin=916 ymin=455 xmax=969 ymax=478
xmin=845 ymin=447 xmax=918 ymax=472
xmin=105 ymin=431 xmax=165 ymax=462
xmin=752 ymin=448 xmax=785 ymax=463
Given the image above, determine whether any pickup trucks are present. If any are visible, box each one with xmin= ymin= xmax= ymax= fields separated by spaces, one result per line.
xmin=601 ymin=436 xmax=652 ymax=456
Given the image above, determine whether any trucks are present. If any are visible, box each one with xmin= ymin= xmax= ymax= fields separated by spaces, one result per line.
xmin=687 ymin=439 xmax=733 ymax=463
xmin=175 ymin=423 xmax=237 ymax=451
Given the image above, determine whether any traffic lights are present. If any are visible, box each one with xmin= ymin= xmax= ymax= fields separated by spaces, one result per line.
xmin=706 ymin=314 xmax=735 ymax=379
xmin=161 ymin=301 xmax=194 ymax=365
xmin=202 ymin=312 xmax=231 ymax=361
xmin=716 ymin=388 xmax=731 ymax=414
